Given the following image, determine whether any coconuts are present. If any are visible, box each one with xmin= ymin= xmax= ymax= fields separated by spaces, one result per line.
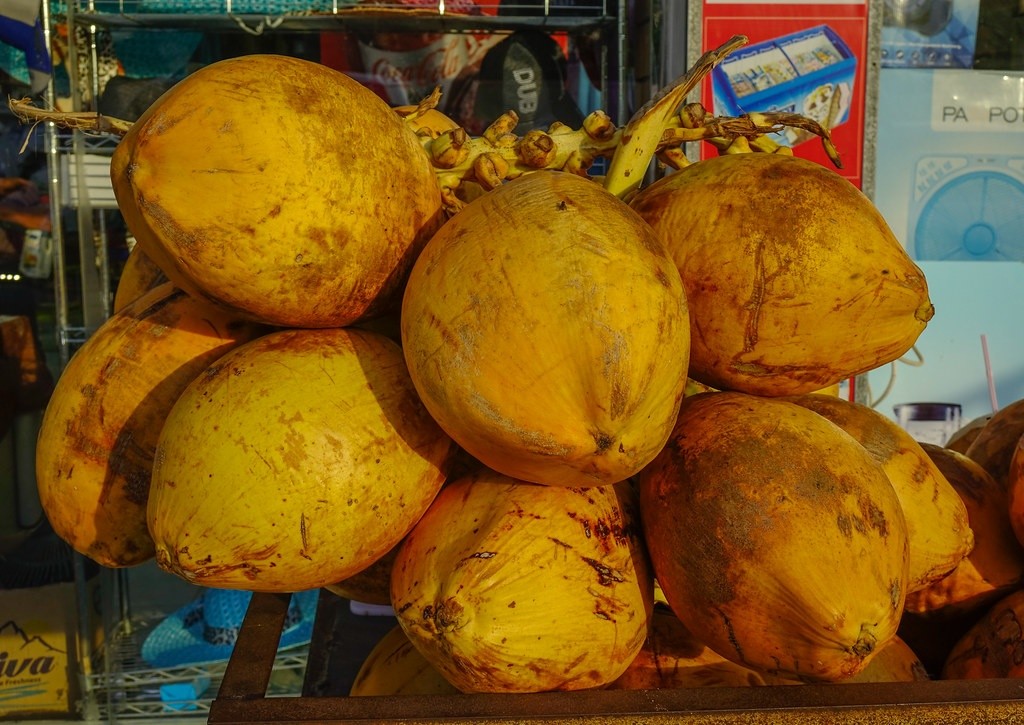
xmin=33 ymin=51 xmax=1024 ymax=695
